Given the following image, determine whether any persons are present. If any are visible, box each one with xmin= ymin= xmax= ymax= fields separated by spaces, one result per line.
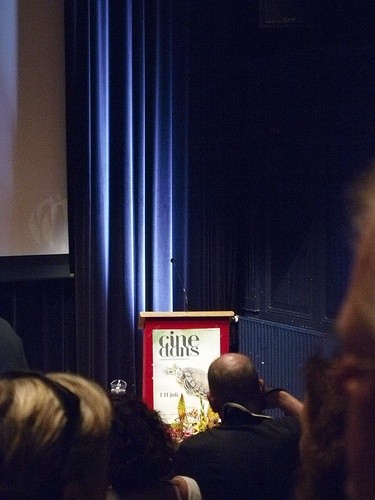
xmin=0 ymin=353 xmax=344 ymax=500
